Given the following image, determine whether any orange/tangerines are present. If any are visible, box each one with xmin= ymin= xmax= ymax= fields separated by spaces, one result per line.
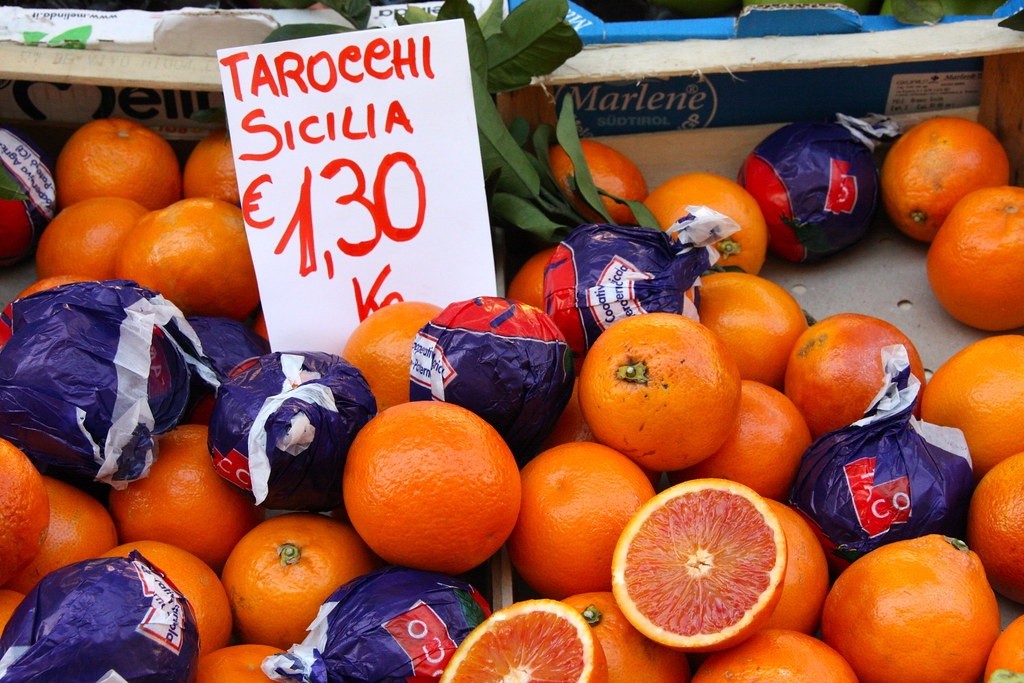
xmin=0 ymin=118 xmax=1023 ymax=683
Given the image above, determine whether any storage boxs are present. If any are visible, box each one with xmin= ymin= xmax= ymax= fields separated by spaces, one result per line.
xmin=0 ymin=0 xmax=1024 ymax=193
